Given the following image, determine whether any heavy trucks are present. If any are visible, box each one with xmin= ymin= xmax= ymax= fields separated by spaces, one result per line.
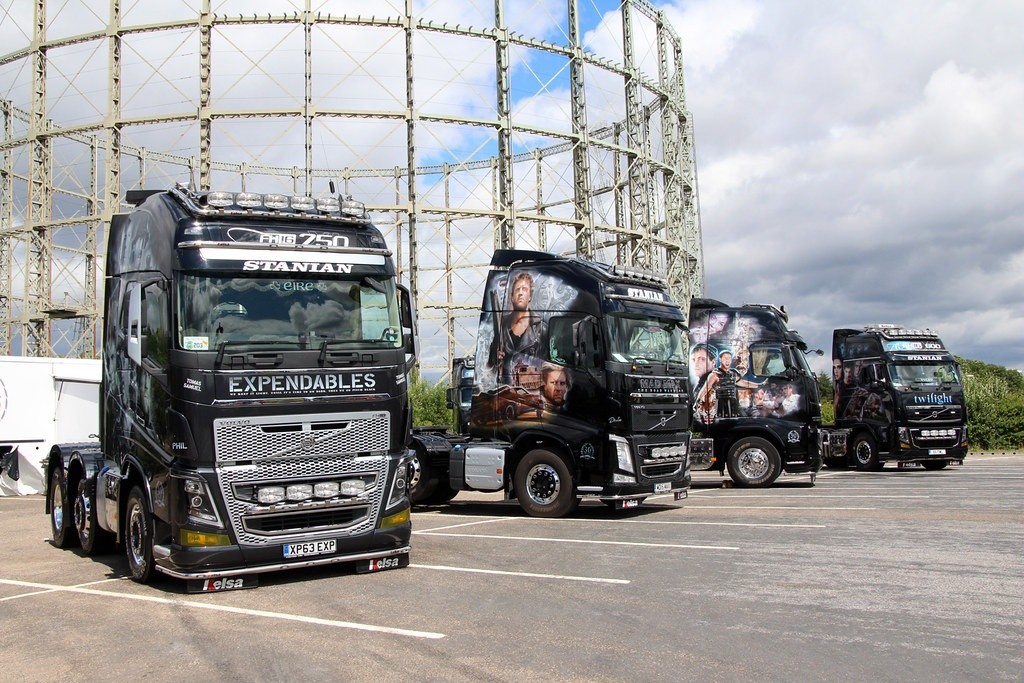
xmin=43 ymin=180 xmax=416 ymax=587
xmin=817 ymin=322 xmax=973 ymax=473
xmin=444 ymin=356 xmax=474 ymax=432
xmin=396 ymin=247 xmax=699 ymax=519
xmin=674 ymin=295 xmax=828 ymax=488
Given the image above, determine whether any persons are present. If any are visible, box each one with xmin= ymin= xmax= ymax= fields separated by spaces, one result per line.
xmin=489 ymin=275 xmax=568 ymax=407
xmin=832 ymin=343 xmax=893 ymax=422
xmin=103 ymin=214 xmax=166 ymax=466
xmin=692 ymin=312 xmax=801 ymax=423
